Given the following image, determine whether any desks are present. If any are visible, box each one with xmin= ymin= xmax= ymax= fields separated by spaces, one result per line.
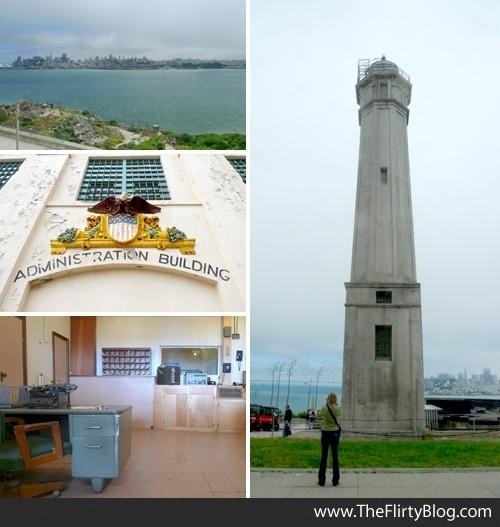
xmin=0 ymin=405 xmax=133 ymax=494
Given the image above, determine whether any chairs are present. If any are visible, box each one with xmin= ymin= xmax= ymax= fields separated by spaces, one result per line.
xmin=0 ymin=411 xmax=72 ymax=499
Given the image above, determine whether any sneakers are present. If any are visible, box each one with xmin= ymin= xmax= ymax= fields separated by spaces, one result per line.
xmin=332 ymin=478 xmax=339 ymax=485
xmin=318 ymin=478 xmax=325 ymax=485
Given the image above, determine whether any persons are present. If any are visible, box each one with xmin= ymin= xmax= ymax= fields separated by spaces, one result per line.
xmin=283 ymin=404 xmax=292 ymax=437
xmin=317 ymin=392 xmax=344 ymax=485
xmin=305 ymin=408 xmax=310 ymax=428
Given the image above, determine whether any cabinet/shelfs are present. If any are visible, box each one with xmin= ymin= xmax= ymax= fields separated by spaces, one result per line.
xmin=101 ymin=347 xmax=152 ymax=376
xmin=155 ymin=386 xmax=217 ymax=433
xmin=218 ymin=399 xmax=245 ymax=433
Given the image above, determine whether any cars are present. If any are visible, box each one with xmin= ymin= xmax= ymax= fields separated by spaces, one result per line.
xmin=251 ymin=407 xmax=280 ymax=431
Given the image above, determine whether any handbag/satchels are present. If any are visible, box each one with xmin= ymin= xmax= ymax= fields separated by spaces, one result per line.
xmin=339 ymin=425 xmax=342 ymax=437
